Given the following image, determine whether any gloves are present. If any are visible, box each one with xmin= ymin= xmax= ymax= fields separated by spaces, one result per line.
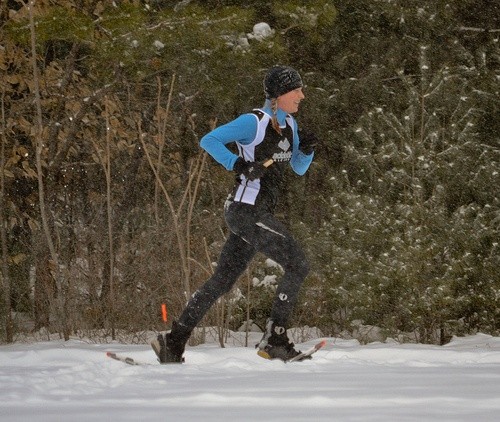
xmin=233 ymin=156 xmax=267 ymax=182
xmin=298 ymin=133 xmax=318 ymax=155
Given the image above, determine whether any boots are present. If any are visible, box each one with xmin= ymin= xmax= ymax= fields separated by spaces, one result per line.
xmin=257 ymin=318 xmax=312 ymax=361
xmin=150 ymin=320 xmax=190 ymax=363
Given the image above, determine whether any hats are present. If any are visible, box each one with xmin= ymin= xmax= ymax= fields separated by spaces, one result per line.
xmin=263 ymin=65 xmax=304 ymax=99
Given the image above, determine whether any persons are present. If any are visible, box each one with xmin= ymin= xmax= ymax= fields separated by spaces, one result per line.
xmin=151 ymin=65 xmax=319 ymax=365
